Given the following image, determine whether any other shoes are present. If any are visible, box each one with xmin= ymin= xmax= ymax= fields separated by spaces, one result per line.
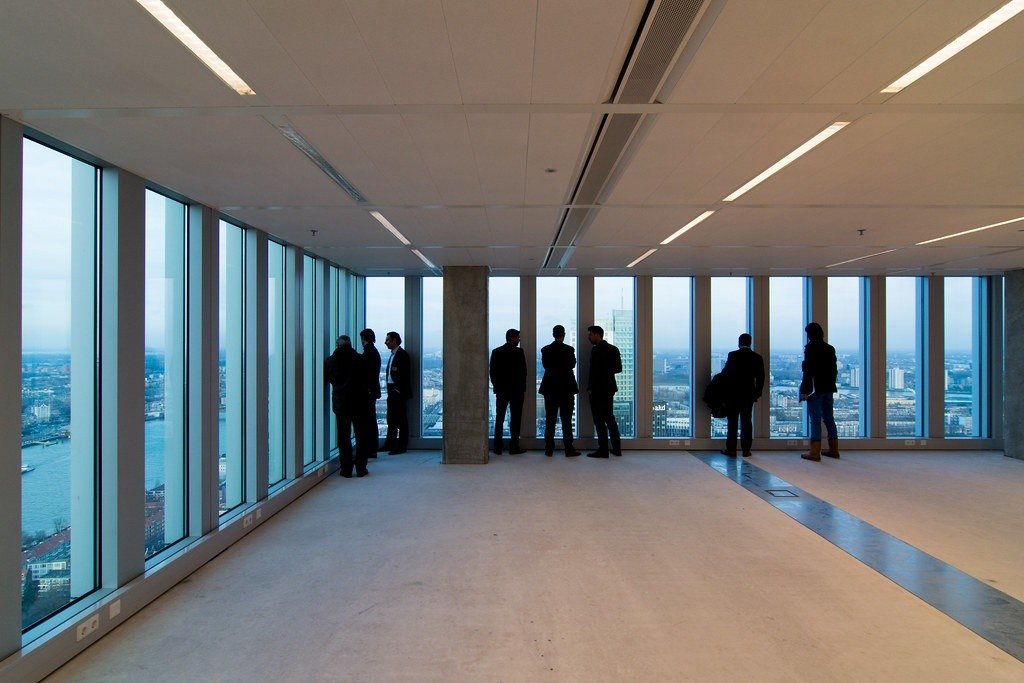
xmin=545 ymin=449 xmax=553 ymax=456
xmin=388 ymin=447 xmax=408 ymax=455
xmin=742 ymin=450 xmax=751 ymax=457
xmin=509 ymin=447 xmax=528 ymax=455
xmin=366 ymin=451 xmax=377 ymax=458
xmin=340 ymin=469 xmax=352 ymax=478
xmin=586 ymin=451 xmax=609 ymax=458
xmin=610 ymin=448 xmax=621 ymax=456
xmin=378 ymin=445 xmax=391 ymax=452
xmin=493 ymin=448 xmax=503 ymax=455
xmin=721 ymin=449 xmax=736 ymax=457
xmin=565 ymin=450 xmax=581 ymax=457
xmin=356 ymin=468 xmax=368 ymax=477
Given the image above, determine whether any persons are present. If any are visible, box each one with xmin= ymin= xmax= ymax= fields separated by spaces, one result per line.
xmin=720 ymin=334 xmax=765 ymax=457
xmin=379 ymin=332 xmax=416 ymax=455
xmin=490 ymin=329 xmax=528 ymax=455
xmin=587 ymin=325 xmax=622 ymax=458
xmin=324 ymin=335 xmax=370 ymax=477
xmin=359 ymin=329 xmax=381 ymax=459
xmin=538 ymin=325 xmax=582 ymax=458
xmin=801 ymin=322 xmax=840 ymax=461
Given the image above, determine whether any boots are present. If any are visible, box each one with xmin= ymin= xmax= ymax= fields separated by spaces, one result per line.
xmin=801 ymin=441 xmax=821 ymax=461
xmin=821 ymin=440 xmax=840 ymax=458
